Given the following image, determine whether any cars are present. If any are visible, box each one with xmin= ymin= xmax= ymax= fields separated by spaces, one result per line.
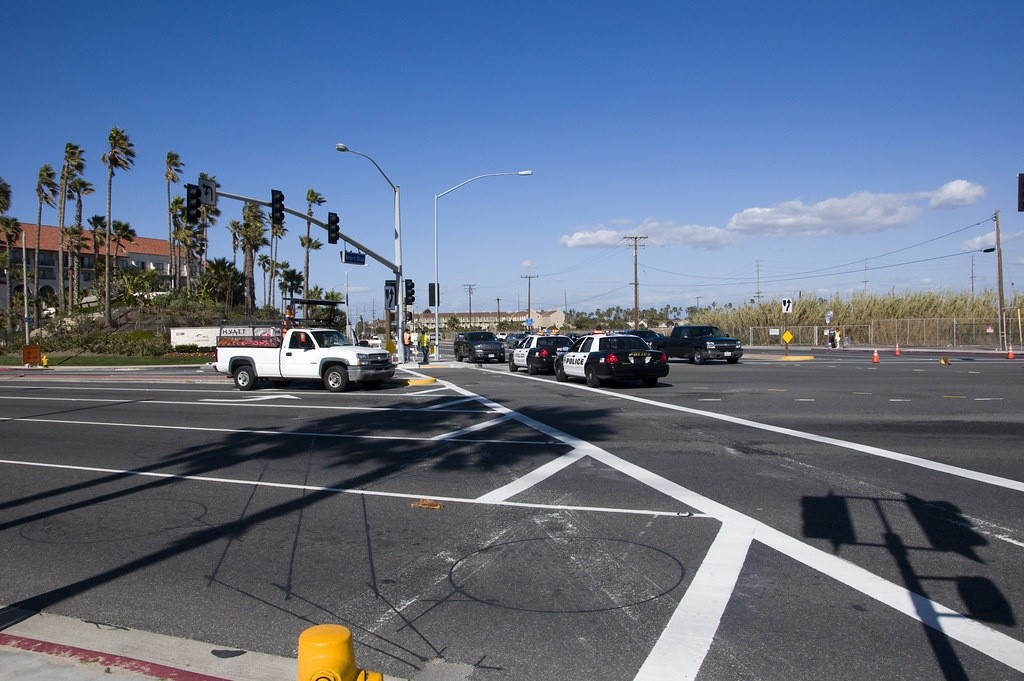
xmin=509 ymin=334 xmax=669 ymax=388
xmin=366 ymin=335 xmax=380 ymax=346
xmin=431 ymin=333 xmax=441 ymax=340
xmin=494 ymin=332 xmax=527 ymax=348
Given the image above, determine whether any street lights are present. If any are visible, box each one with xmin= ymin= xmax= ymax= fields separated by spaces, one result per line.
xmin=335 ymin=142 xmax=405 ymax=364
xmin=433 ymin=170 xmax=533 ymax=362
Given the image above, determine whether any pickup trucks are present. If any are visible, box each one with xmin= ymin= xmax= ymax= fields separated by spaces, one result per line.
xmin=454 ymin=332 xmax=506 ymax=363
xmin=206 ymin=319 xmax=395 ymax=393
xmin=652 ymin=326 xmax=743 ymax=365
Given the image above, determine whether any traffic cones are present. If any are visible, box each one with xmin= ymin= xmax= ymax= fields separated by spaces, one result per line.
xmin=893 ymin=343 xmax=901 ymax=355
xmin=872 ymin=348 xmax=881 ymax=363
xmin=1006 ymin=345 xmax=1015 ymax=359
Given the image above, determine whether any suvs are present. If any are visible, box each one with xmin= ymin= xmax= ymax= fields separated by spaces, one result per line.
xmin=626 ymin=330 xmax=664 ymax=346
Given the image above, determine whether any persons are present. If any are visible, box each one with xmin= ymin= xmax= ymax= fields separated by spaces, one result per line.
xmin=367 ymin=329 xmax=431 ymax=364
xmin=835 ymin=328 xmax=841 ymax=348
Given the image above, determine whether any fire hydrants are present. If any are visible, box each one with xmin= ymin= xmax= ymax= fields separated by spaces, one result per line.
xmin=297 ymin=623 xmax=383 ymax=681
xmin=41 ymin=355 xmax=49 ymax=368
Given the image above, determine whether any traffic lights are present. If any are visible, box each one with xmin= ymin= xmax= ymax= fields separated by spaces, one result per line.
xmin=328 ymin=212 xmax=339 ymax=244
xmin=405 ymin=279 xmax=415 ymax=305
xmin=186 ymin=183 xmax=202 ymax=223
xmin=271 ymin=189 xmax=284 ymax=224
